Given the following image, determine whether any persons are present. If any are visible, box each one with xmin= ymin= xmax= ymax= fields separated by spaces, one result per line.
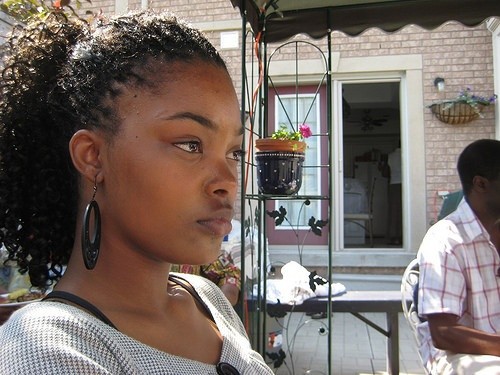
xmin=388 ymin=139 xmax=402 ymax=244
xmin=417 ymin=139 xmax=500 ymax=375
xmin=0 ymin=9 xmax=275 ymax=375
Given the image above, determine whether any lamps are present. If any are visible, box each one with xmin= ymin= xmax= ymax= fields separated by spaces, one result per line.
xmin=361 ymin=125 xmax=374 ymax=130
xmin=434 ymin=77 xmax=445 ymax=91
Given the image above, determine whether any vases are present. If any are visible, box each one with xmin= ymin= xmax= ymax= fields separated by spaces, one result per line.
xmin=256 ymin=140 xmax=304 ymax=196
xmin=428 ymin=101 xmax=487 ymax=125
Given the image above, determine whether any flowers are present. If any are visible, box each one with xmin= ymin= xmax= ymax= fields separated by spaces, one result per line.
xmin=443 ymin=88 xmax=496 ymax=119
xmin=270 ymin=123 xmax=311 ymax=140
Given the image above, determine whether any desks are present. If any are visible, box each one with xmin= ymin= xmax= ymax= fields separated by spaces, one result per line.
xmin=246 ymin=291 xmax=414 ymax=375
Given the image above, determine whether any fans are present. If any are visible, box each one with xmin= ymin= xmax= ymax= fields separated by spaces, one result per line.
xmin=348 ymin=109 xmax=388 ymax=126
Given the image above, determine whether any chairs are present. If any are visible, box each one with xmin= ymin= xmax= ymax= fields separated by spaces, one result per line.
xmin=400 ymin=258 xmax=440 ymax=375
xmin=344 ymin=177 xmax=377 ymax=247
